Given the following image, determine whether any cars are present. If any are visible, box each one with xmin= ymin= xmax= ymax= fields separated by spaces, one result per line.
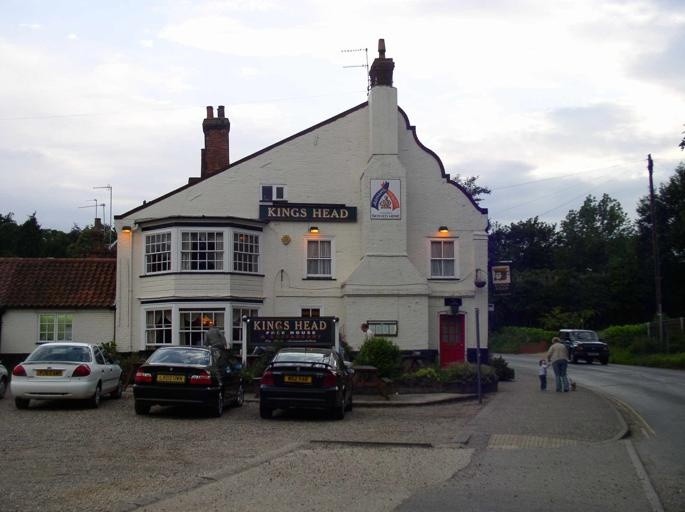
xmin=9 ymin=341 xmax=125 ymax=408
xmin=131 ymin=345 xmax=246 ymax=419
xmin=258 ymin=347 xmax=356 ymax=421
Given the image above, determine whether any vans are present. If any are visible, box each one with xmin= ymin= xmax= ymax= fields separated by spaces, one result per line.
xmin=558 ymin=329 xmax=610 ymax=366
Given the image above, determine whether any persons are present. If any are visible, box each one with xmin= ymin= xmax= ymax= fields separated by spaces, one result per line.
xmin=538 ymin=360 xmax=551 ymax=391
xmin=547 ymin=336 xmax=569 ymax=392
xmin=361 ymin=324 xmax=374 ymax=348
xmin=204 ymin=322 xmax=226 ymax=351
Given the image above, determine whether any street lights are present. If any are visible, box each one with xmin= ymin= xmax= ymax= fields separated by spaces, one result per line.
xmin=77 ymin=184 xmax=113 ymax=245
xmin=473 ymin=266 xmax=488 ymax=405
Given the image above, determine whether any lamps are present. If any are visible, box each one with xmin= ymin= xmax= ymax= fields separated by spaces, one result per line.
xmin=122 ymin=225 xmax=131 ymax=232
xmin=439 ymin=226 xmax=447 ymax=232
xmin=310 ymin=227 xmax=318 ymax=233
